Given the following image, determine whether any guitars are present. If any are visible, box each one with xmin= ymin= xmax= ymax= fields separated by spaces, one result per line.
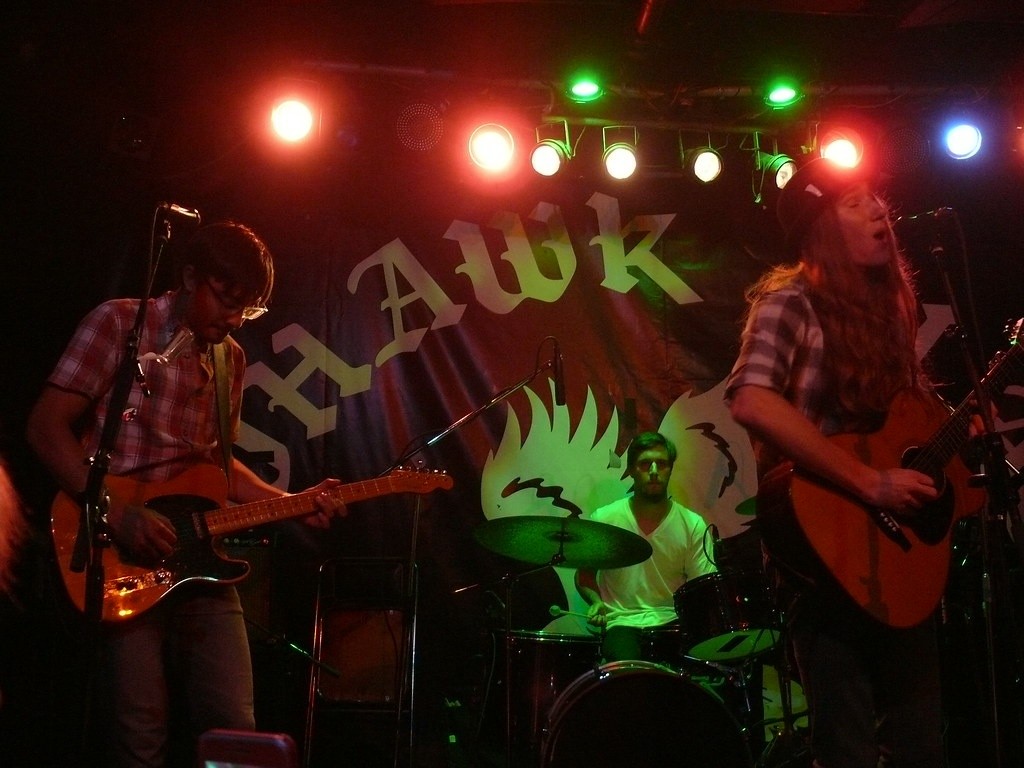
xmin=756 ymin=316 xmax=1024 ymax=638
xmin=48 ymin=462 xmax=456 ymax=624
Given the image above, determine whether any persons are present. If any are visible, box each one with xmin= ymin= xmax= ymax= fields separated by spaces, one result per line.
xmin=720 ymin=158 xmax=1000 ymax=768
xmin=574 ymin=431 xmax=719 ymax=662
xmin=26 ymin=221 xmax=349 ymax=768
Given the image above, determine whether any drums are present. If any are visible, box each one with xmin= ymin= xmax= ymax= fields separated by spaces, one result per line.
xmin=671 ymin=572 xmax=780 ymax=661
xmin=639 ymin=624 xmax=726 ymax=684
xmin=307 ymin=601 xmax=411 ymax=718
xmin=493 ymin=626 xmax=604 ymax=718
xmin=537 ymin=658 xmax=756 ymax=767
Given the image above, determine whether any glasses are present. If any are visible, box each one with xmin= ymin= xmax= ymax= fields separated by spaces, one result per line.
xmin=192 ymin=273 xmax=268 ymax=320
xmin=629 ymin=458 xmax=672 ymax=470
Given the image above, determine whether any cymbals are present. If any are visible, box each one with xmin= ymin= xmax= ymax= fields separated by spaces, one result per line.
xmin=471 ymin=515 xmax=655 ymax=574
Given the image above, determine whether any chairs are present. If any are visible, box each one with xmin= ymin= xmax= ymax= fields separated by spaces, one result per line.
xmin=301 ymin=553 xmax=421 ymax=768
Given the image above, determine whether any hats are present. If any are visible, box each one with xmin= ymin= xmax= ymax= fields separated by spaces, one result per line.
xmin=775 ymin=158 xmax=877 ymax=256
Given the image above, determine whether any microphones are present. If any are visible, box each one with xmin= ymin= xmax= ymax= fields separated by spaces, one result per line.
xmin=160 ymin=204 xmax=201 ymax=227
xmin=553 ymin=340 xmax=566 ymax=406
xmin=712 ymin=526 xmax=722 ymax=565
xmin=893 ymin=207 xmax=953 ymax=235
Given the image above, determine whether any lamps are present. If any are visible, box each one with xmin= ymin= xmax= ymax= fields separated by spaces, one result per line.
xmin=763 ymin=84 xmax=807 ymax=111
xmin=602 ymin=125 xmax=641 ymax=181
xmin=563 ymin=67 xmax=608 ymax=108
xmin=741 ymin=132 xmax=799 ymax=203
xmin=677 ymin=127 xmax=725 ymax=186
xmin=528 ymin=119 xmax=573 ymax=179
